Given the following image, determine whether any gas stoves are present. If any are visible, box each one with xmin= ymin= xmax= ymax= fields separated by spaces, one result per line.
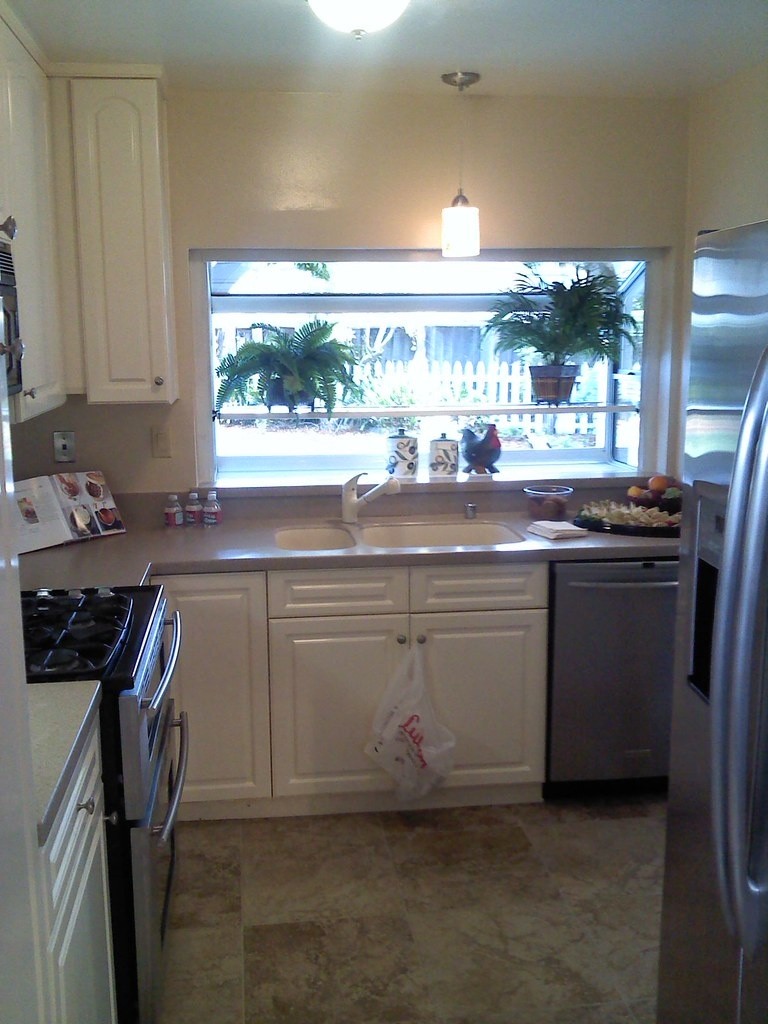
xmin=21 ymin=584 xmax=166 ymax=690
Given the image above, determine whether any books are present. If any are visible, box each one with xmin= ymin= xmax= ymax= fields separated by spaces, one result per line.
xmin=527 ymin=519 xmax=588 ymax=542
xmin=15 ymin=471 xmax=126 ymax=555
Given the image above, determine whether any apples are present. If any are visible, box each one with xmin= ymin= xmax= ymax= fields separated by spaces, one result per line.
xmin=648 ymin=476 xmax=668 ymax=493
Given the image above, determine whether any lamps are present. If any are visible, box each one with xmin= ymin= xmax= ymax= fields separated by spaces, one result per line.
xmin=442 ymin=72 xmax=481 ymax=258
xmin=307 ymin=0 xmax=411 ymax=41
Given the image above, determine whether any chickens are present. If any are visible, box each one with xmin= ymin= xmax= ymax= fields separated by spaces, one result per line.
xmin=459 ymin=424 xmax=501 ymax=474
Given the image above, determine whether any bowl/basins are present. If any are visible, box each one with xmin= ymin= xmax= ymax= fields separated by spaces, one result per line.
xmin=627 ymin=485 xmax=683 ymax=515
xmin=524 ymin=486 xmax=573 ymax=518
xmin=70 ymin=508 xmax=91 ymax=534
xmin=98 ymin=509 xmax=116 ymax=525
xmin=59 ymin=481 xmax=81 ymax=498
xmin=85 ymin=481 xmax=103 ymax=500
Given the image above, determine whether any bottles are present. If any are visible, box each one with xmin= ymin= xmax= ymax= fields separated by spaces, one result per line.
xmin=387 ymin=429 xmax=418 ymax=478
xmin=163 ymin=491 xmax=223 ymax=527
xmin=429 ymin=433 xmax=458 ymax=477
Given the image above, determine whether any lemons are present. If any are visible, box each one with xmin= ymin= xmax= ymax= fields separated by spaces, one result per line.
xmin=627 ymin=485 xmax=641 ymax=497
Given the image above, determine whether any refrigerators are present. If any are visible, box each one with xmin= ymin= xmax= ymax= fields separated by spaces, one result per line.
xmin=657 ymin=218 xmax=767 ymax=1022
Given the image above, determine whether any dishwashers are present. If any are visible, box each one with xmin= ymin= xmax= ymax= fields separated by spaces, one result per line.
xmin=549 ymin=557 xmax=681 ymax=789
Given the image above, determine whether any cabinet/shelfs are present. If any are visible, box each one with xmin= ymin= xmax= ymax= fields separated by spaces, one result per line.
xmin=0 ymin=18 xmax=179 ymax=426
xmin=150 ymin=562 xmax=551 ymax=822
xmin=38 ymin=707 xmax=118 ymax=1024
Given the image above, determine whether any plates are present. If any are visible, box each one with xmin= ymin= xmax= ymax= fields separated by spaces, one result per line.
xmin=575 ymin=510 xmax=683 ymax=538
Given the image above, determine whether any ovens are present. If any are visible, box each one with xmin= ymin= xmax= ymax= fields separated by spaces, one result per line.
xmin=98 ymin=599 xmax=190 ymax=1024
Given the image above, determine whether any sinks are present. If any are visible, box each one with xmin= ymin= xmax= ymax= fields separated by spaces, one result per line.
xmin=359 ymin=523 xmax=526 ymax=545
xmin=275 ymin=529 xmax=356 ymax=550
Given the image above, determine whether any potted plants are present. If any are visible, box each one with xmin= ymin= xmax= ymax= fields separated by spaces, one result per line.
xmin=479 ymin=260 xmax=638 ymax=406
xmin=214 ymin=320 xmax=360 ymax=419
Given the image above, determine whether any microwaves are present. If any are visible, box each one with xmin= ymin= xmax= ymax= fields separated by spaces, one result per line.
xmin=0 ymin=238 xmax=22 ymax=395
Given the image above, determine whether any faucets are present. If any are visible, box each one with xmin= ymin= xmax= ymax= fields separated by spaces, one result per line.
xmin=341 ymin=471 xmax=400 ymax=523
